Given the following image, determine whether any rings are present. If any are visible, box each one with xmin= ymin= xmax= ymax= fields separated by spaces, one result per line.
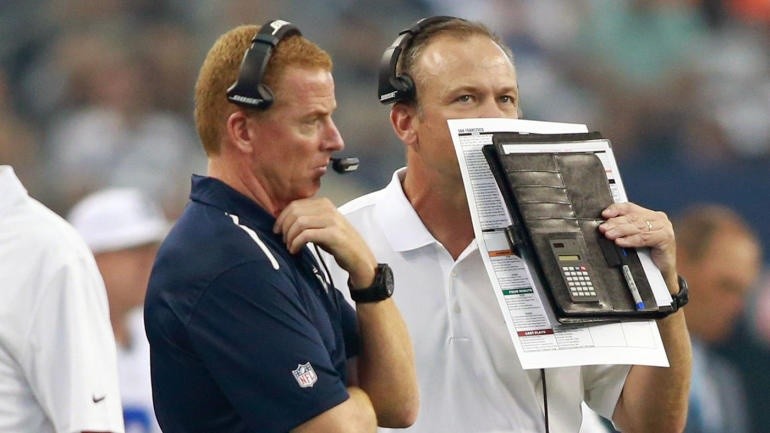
xmin=646 ymin=221 xmax=651 ymax=232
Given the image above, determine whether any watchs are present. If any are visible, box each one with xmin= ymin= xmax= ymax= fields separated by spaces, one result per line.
xmin=347 ymin=263 xmax=395 ymax=303
xmin=671 ymin=274 xmax=689 ymax=307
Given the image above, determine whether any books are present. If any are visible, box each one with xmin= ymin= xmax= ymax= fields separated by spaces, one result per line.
xmin=482 ymin=130 xmax=676 ymax=324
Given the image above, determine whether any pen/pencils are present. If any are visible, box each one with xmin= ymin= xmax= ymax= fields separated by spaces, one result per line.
xmin=620 ymin=247 xmax=645 ymax=310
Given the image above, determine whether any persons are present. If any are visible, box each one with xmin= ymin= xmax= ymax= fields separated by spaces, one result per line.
xmin=599 ymin=200 xmax=760 ymax=433
xmin=143 ymin=26 xmax=420 ymax=433
xmin=0 ymin=166 xmax=125 ymax=433
xmin=67 ymin=186 xmax=162 ymax=433
xmin=306 ymin=17 xmax=693 ymax=433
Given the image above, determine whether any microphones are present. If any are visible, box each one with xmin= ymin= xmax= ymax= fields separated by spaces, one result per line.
xmin=330 ymin=156 xmax=360 ymax=174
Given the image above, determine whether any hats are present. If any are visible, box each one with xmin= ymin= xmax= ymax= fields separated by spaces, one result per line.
xmin=66 ymin=187 xmax=175 ymax=256
